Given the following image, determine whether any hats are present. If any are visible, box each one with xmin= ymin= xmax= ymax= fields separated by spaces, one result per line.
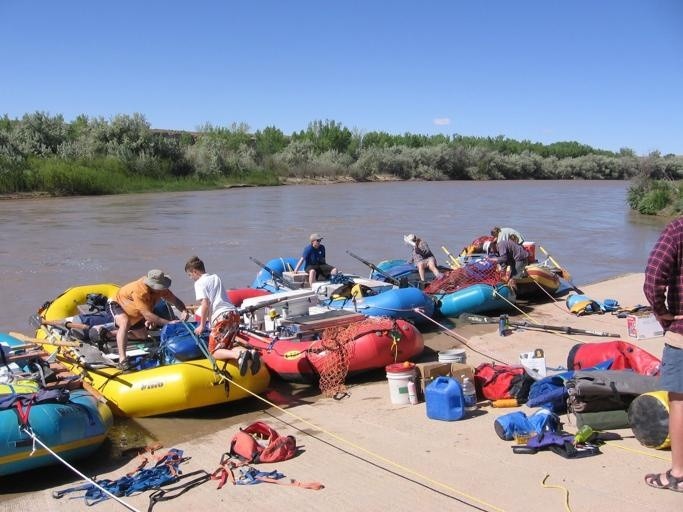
xmin=403 ymin=233 xmax=416 ymax=246
xmin=310 ymin=234 xmax=323 ymax=241
xmin=145 ymin=270 xmax=172 ymax=291
xmin=483 ymin=240 xmax=492 ymax=256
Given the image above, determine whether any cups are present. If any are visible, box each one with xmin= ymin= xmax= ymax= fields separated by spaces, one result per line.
xmin=515 ymin=432 xmax=530 ymax=443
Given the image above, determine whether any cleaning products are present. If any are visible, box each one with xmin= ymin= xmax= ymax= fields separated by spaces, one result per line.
xmin=499 ymin=314 xmax=509 ymax=336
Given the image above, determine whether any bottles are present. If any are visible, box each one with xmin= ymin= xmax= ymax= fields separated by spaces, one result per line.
xmin=572 ymin=425 xmax=593 ymax=447
xmin=407 ymin=380 xmax=418 ymax=405
xmin=499 ymin=314 xmax=506 ymax=337
xmin=506 ymin=316 xmax=509 ymax=329
xmin=490 ymin=398 xmax=518 ymax=408
xmin=462 ymin=377 xmax=477 ymax=412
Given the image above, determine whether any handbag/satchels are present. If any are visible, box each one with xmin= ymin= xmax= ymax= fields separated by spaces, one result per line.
xmin=230 ymin=420 xmax=297 ymax=465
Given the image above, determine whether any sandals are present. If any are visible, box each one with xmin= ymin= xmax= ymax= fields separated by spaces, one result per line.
xmin=237 ymin=349 xmax=261 ymax=376
xmin=645 ymin=471 xmax=682 ymax=493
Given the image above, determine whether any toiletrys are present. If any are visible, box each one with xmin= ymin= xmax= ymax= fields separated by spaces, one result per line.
xmin=407 ymin=377 xmax=418 ymax=405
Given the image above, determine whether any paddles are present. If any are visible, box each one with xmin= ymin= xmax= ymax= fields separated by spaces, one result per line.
xmin=538 ymin=245 xmax=584 ymax=295
xmin=28 ymin=314 xmax=90 ymax=331
xmin=9 ymin=332 xmax=83 ymax=348
xmin=458 ymin=312 xmax=621 ymax=338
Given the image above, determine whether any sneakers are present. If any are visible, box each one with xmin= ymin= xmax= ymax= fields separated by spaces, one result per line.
xmin=117 ymin=360 xmax=130 ymax=370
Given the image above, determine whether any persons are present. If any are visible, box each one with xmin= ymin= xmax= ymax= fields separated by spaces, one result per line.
xmin=102 ymin=266 xmax=188 ymax=374
xmin=490 ymin=226 xmax=524 ymax=245
xmin=508 ymin=234 xmax=519 ymax=245
xmin=482 ymin=239 xmax=530 ymax=283
xmin=183 ymin=256 xmax=260 ymax=376
xmin=403 ymin=233 xmax=442 ymax=283
xmin=465 ymin=235 xmax=496 ymax=255
xmin=292 ymin=233 xmax=337 ymax=288
xmin=644 ymin=213 xmax=683 ymax=493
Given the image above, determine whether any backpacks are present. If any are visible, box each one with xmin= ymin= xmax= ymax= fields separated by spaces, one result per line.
xmin=473 ymin=362 xmax=533 ymax=405
xmin=418 ymin=239 xmax=430 ymax=254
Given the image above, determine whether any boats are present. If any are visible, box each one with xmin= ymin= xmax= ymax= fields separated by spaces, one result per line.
xmin=368 ymin=258 xmax=519 ymax=319
xmin=193 ymin=287 xmax=425 ymax=384
xmin=35 ymin=283 xmax=271 ymax=418
xmin=253 ymin=257 xmax=437 ymax=328
xmin=460 ymin=244 xmax=562 ymax=296
xmin=0 ymin=331 xmax=113 ymax=479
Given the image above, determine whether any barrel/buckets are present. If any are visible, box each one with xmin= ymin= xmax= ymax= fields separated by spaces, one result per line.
xmin=425 ymin=376 xmax=464 ymax=421
xmin=522 ymin=241 xmax=535 ymax=263
xmin=385 ymin=362 xmax=417 ymax=404
xmin=462 ymin=378 xmax=478 ymax=407
xmin=438 ymin=349 xmax=466 ymax=363
